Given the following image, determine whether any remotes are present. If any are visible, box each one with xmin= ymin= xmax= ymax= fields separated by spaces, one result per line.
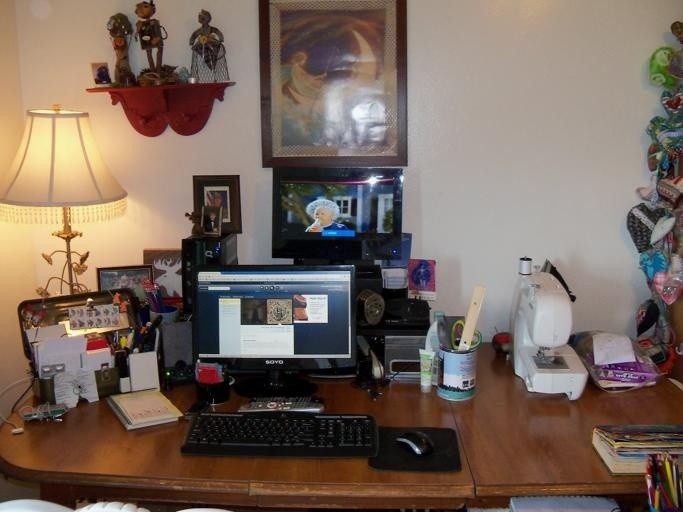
xmin=239 ymin=401 xmax=325 ymax=416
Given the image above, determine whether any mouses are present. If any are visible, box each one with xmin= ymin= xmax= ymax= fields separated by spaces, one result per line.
xmin=395 ymin=431 xmax=435 ymax=458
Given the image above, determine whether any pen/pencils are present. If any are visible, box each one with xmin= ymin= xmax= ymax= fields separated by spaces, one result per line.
xmin=143 ymin=280 xmax=164 ymax=312
xmin=645 ymin=450 xmax=683 ymax=512
xmin=113 ymin=307 xmax=163 ymax=353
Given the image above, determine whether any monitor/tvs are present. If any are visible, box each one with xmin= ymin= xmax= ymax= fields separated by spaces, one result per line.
xmin=196 ymin=268 xmax=352 ymax=397
xmin=271 ymin=167 xmax=403 ymax=266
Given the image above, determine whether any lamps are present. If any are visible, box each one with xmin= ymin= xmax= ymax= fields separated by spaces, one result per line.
xmin=0 ymin=102 xmax=129 ymax=294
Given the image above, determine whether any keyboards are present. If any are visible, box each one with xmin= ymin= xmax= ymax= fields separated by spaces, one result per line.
xmin=180 ymin=412 xmax=379 ymax=456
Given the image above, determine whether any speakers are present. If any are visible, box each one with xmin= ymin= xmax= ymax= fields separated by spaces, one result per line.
xmin=163 ymin=322 xmax=192 ymax=379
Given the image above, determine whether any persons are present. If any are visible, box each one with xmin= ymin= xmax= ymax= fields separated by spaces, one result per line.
xmin=205 ymin=210 xmax=219 ymax=233
xmin=304 ymin=199 xmax=349 ymax=232
xmin=212 ymin=192 xmax=228 ymax=220
xmin=293 ymin=294 xmax=309 ymax=322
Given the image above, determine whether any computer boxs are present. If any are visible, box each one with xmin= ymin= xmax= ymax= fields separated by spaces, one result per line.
xmin=183 ymin=232 xmax=238 ymax=325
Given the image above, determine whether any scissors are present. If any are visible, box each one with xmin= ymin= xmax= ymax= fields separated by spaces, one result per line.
xmin=451 ymin=321 xmax=482 ymax=351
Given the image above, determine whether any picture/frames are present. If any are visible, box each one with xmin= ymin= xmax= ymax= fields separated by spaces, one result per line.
xmin=96 ymin=263 xmax=154 ymax=293
xmin=190 ymin=174 xmax=243 ymax=235
xmin=255 ymin=0 xmax=409 ymax=170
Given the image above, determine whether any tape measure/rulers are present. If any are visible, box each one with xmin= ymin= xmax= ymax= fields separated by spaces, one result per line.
xmin=457 ymin=285 xmax=486 ymax=351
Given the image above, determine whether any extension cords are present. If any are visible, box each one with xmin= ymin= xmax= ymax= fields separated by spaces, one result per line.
xmin=354 ymin=332 xmax=386 ymax=381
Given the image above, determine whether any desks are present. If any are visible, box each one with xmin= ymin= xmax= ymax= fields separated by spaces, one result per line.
xmin=0 ymin=338 xmax=683 ymax=510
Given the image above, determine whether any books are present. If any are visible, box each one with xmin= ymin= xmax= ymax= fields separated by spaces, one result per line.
xmin=105 ymin=388 xmax=179 ymax=430
xmin=110 ymin=388 xmax=185 ymax=425
xmin=509 ymin=495 xmax=621 ymax=512
xmin=591 ymin=423 xmax=683 ymax=476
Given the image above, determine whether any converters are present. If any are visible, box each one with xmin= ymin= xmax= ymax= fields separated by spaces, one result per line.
xmin=357 ymin=360 xmax=374 ymax=391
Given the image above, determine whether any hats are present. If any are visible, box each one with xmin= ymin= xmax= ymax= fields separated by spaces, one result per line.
xmin=306 ymin=199 xmax=340 ymax=220
